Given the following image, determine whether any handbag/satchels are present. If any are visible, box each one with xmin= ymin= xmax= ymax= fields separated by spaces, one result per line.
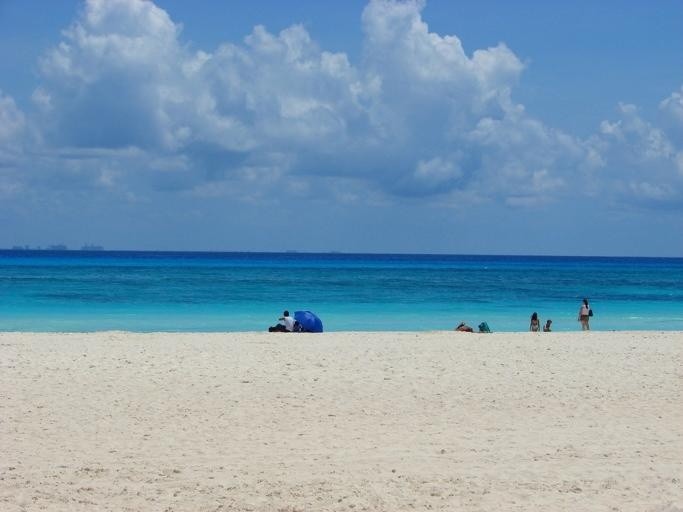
xmin=588 ymin=310 xmax=593 ymax=316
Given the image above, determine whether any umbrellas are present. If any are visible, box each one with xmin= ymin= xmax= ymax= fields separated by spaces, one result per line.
xmin=293 ymin=309 xmax=323 ymax=332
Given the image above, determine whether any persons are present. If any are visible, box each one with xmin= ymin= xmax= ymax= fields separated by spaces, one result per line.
xmin=577 ymin=298 xmax=589 ymax=331
xmin=529 ymin=312 xmax=540 ymax=332
xmin=542 ymin=319 xmax=552 ymax=332
xmin=268 ymin=310 xmax=294 ymax=332
